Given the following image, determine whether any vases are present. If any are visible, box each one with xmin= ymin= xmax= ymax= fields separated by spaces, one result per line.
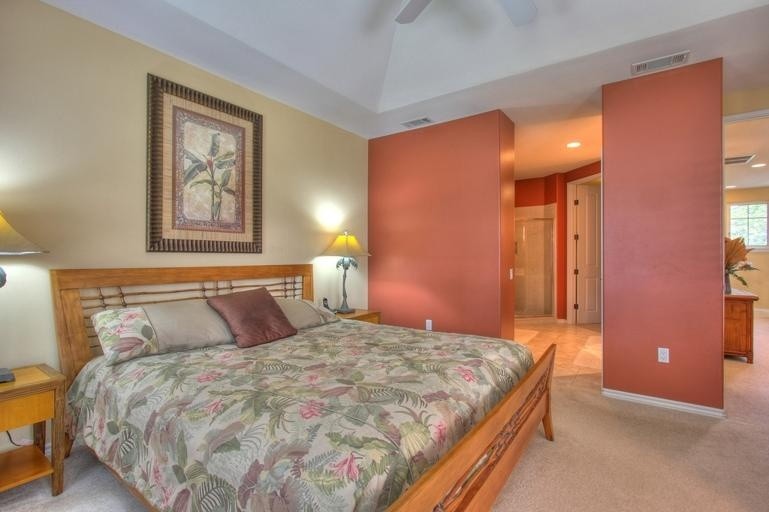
xmin=725 ymin=272 xmax=731 ymax=294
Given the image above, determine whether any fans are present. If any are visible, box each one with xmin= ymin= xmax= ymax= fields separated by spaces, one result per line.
xmin=395 ymin=0 xmax=538 ymax=25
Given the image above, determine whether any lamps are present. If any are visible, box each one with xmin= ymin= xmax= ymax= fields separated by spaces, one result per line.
xmin=0 ymin=211 xmax=49 ymax=383
xmin=323 ymin=232 xmax=372 ymax=314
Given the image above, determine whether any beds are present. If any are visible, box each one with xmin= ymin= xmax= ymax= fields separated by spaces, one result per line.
xmin=50 ymin=264 xmax=556 ymax=512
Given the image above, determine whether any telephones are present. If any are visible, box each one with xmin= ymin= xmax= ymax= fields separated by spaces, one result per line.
xmin=323 ymin=298 xmax=337 ymax=314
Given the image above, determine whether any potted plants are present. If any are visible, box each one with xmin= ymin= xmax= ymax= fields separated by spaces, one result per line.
xmin=725 ymin=237 xmax=760 ymax=285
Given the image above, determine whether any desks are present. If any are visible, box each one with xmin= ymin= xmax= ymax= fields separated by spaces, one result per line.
xmin=724 ymin=288 xmax=759 ymax=364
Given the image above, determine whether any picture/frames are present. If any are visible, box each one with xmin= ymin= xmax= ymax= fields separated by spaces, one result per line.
xmin=147 ymin=73 xmax=262 ymax=253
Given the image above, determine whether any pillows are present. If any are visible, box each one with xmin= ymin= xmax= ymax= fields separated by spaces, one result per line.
xmin=90 ymin=299 xmax=235 ymax=367
xmin=206 ymin=287 xmax=297 ymax=348
xmin=275 ymin=299 xmax=341 ymax=330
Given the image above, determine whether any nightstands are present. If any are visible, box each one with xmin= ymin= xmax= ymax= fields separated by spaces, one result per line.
xmin=336 ymin=309 xmax=382 ymax=324
xmin=0 ymin=364 xmax=67 ymax=497
